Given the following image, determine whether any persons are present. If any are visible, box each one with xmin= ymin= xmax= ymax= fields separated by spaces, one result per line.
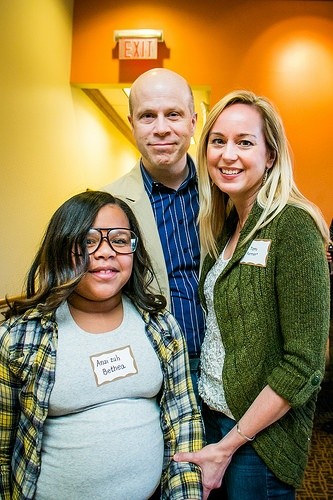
xmin=1 ymin=191 xmax=208 ymax=500
xmin=99 ymin=68 xmax=201 ymax=407
xmin=173 ymin=89 xmax=333 ymax=500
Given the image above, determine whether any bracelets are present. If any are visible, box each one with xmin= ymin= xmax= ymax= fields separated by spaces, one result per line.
xmin=236 ymin=421 xmax=255 ymax=441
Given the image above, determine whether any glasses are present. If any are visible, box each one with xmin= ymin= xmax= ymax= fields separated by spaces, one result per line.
xmin=71 ymin=228 xmax=139 ymax=254
